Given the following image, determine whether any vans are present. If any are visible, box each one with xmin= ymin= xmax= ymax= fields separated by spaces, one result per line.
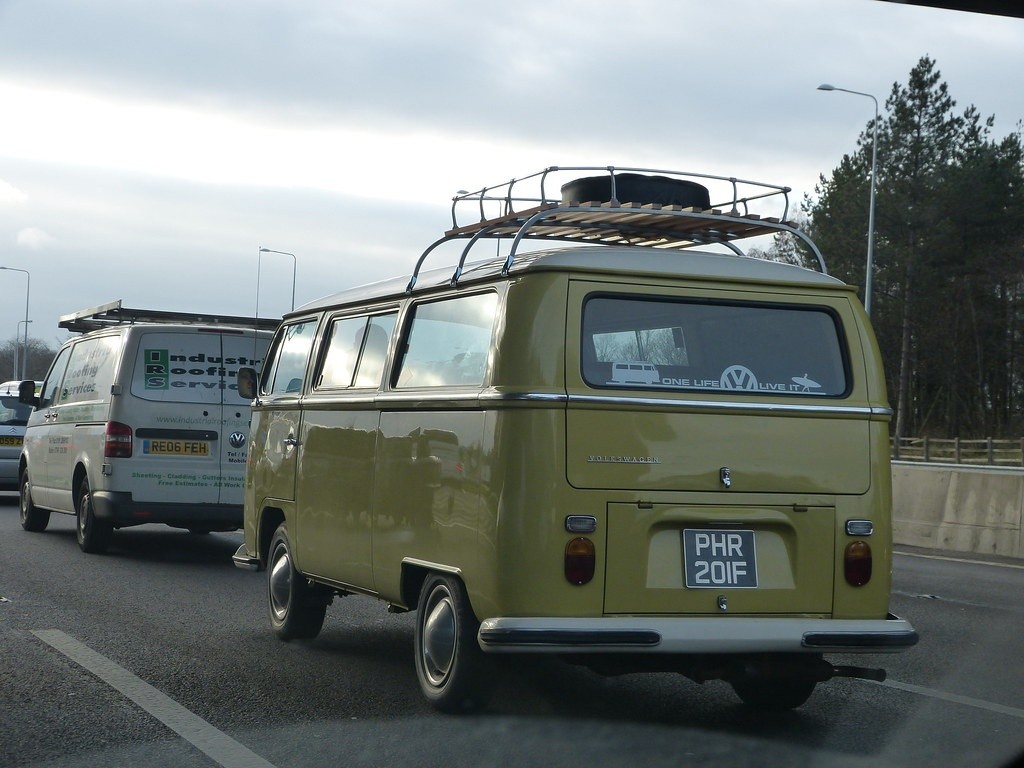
xmin=237 ymin=167 xmax=918 ymax=714
xmin=14 ymin=300 xmax=283 ymax=553
xmin=0 ymin=379 xmax=43 ymax=492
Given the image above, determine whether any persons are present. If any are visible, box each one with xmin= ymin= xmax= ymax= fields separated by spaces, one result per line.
xmin=353 ymin=324 xmax=388 ymax=362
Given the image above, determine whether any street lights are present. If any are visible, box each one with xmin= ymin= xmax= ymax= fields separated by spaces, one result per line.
xmin=14 ymin=320 xmax=34 ymax=382
xmin=0 ymin=266 xmax=30 ymax=380
xmin=259 ymin=248 xmax=297 ymax=312
xmin=817 ymin=82 xmax=880 ymax=321
